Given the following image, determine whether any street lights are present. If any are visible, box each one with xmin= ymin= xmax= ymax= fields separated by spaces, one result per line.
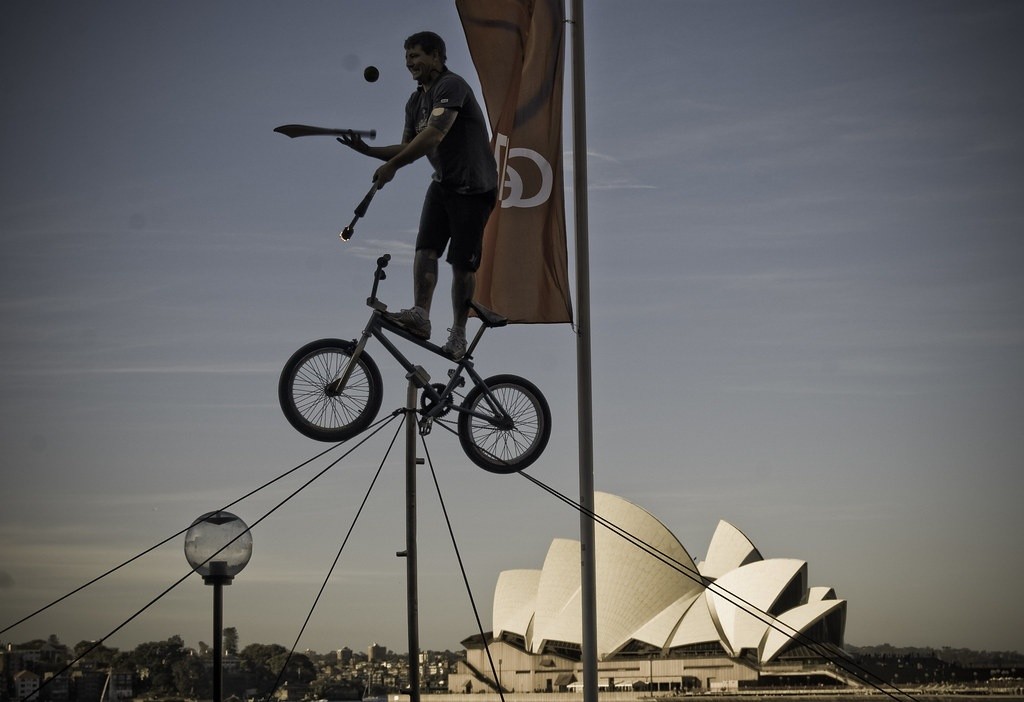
xmin=185 ymin=507 xmax=253 ymax=701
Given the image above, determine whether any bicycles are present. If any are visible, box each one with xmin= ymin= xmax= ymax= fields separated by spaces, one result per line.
xmin=279 ymin=253 xmax=553 ymax=476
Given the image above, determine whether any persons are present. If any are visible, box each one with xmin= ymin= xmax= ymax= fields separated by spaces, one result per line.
xmin=336 ymin=31 xmax=499 ymax=362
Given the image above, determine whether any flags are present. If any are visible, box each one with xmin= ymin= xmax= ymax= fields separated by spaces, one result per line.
xmin=455 ymin=0 xmax=574 ymax=324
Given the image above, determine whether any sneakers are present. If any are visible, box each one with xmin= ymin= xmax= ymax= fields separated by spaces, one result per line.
xmin=442 ymin=327 xmax=467 ymax=361
xmin=382 ymin=307 xmax=431 ymax=339
